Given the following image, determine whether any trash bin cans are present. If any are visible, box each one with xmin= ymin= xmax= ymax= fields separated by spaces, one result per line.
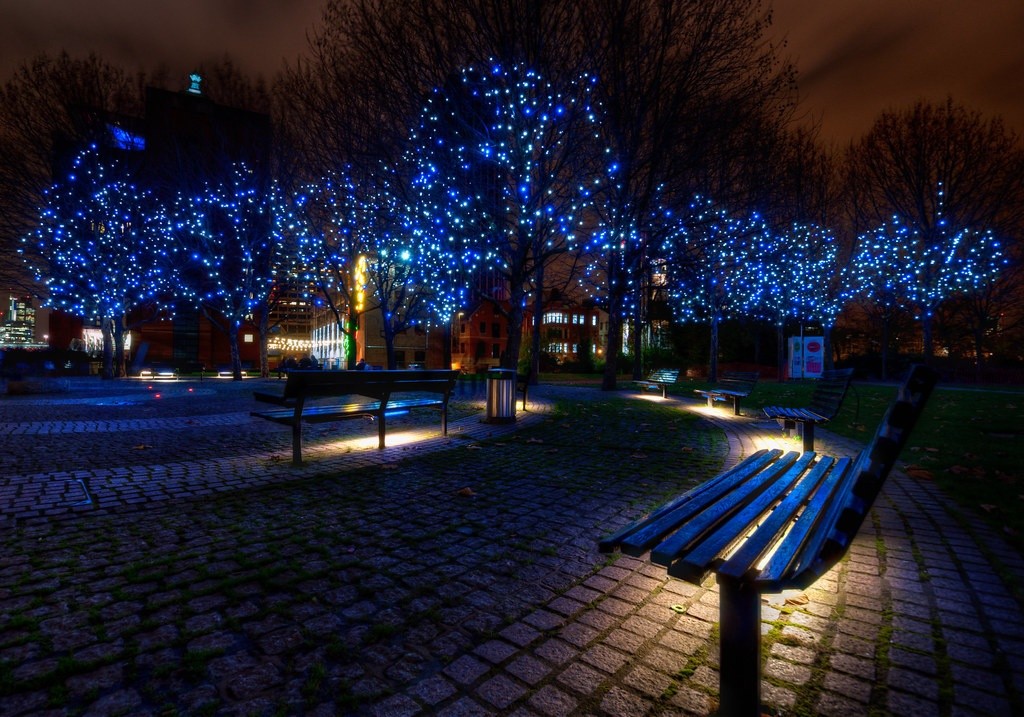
xmin=486 ymin=369 xmax=516 ymax=422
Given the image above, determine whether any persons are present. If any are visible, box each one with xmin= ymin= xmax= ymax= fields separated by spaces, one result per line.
xmin=311 ymin=355 xmax=318 ymax=369
xmin=356 ymin=359 xmax=365 ymax=370
xmin=299 ymin=354 xmax=311 ymax=368
xmin=278 ymin=354 xmax=299 ymax=380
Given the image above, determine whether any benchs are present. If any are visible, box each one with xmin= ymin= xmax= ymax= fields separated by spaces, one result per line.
xmin=598 ymin=360 xmax=936 ymax=717
xmin=249 ymin=370 xmax=457 ymax=465
xmin=307 ymin=364 xmax=324 ymax=370
xmin=372 ymin=366 xmax=383 ymax=370
